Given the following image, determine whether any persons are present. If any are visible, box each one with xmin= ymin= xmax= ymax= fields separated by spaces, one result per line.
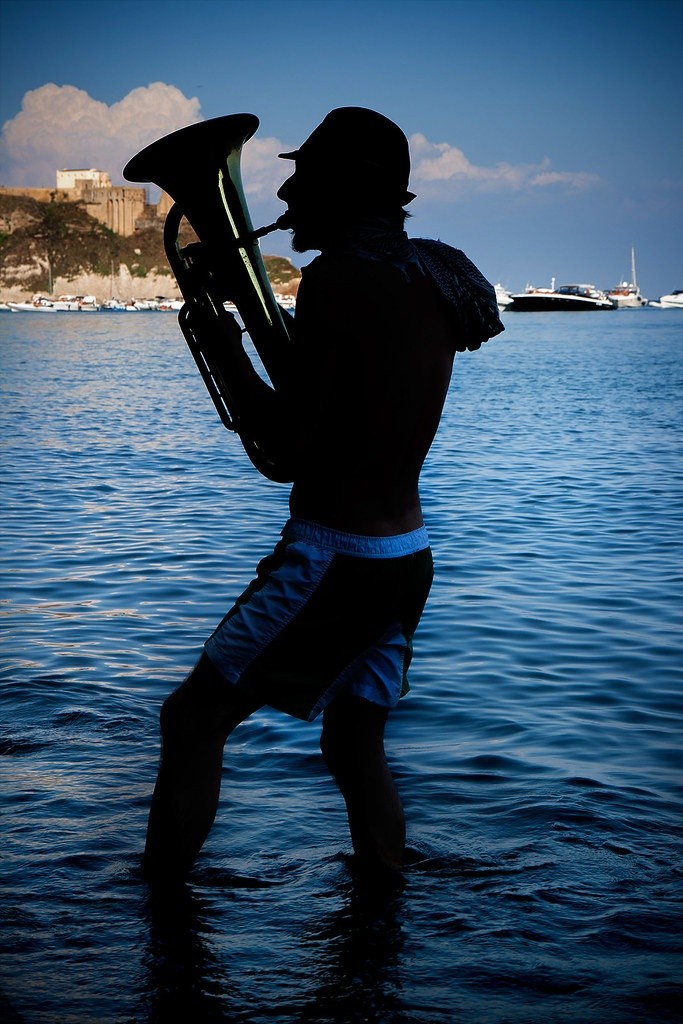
xmin=136 ymin=107 xmax=455 ymax=890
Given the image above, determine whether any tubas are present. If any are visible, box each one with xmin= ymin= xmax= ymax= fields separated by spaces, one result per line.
xmin=121 ymin=111 xmax=306 ymax=482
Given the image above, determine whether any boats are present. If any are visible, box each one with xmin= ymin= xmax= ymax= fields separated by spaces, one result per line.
xmin=494 ymin=284 xmax=515 ymax=313
xmin=509 ymin=284 xmax=614 ymax=310
xmin=648 ymin=290 xmax=683 ymax=309
xmin=0 ymin=295 xmax=295 ymax=314
xmin=607 ymin=243 xmax=648 ymax=310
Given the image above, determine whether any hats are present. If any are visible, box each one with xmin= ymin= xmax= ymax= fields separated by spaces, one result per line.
xmin=278 ymin=106 xmax=411 ymax=188
